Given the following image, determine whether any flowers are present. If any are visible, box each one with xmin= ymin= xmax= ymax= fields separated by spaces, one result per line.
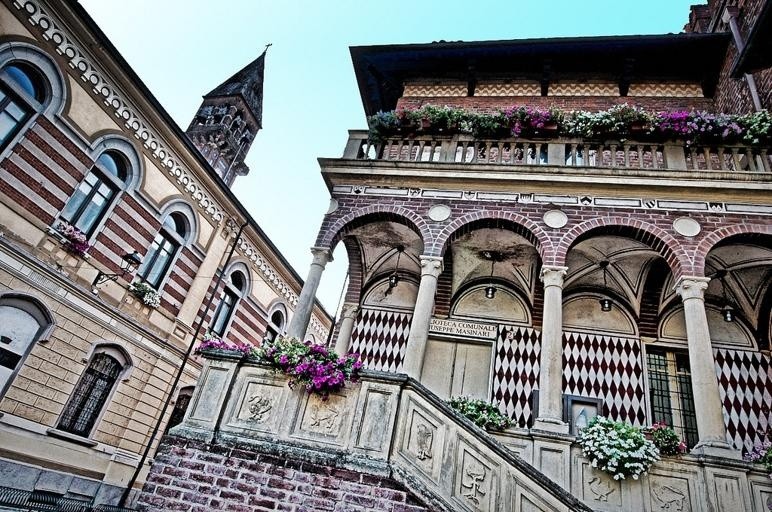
xmin=132 ymin=282 xmax=161 ymax=308
xmin=367 ymin=99 xmax=771 ymax=149
xmin=443 ymin=396 xmax=771 ymax=481
xmin=56 ymin=221 xmax=89 ymax=254
xmin=194 ymin=333 xmax=364 ymax=401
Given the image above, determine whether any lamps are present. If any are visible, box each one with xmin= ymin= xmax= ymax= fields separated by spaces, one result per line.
xmin=389 ymin=244 xmax=736 ymax=322
xmin=91 ymin=251 xmax=143 ymax=286
